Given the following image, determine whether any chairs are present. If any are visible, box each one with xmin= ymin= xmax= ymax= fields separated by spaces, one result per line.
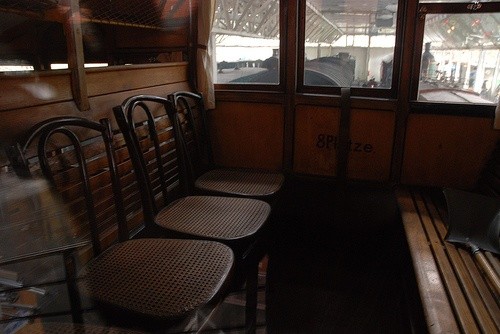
xmin=8 ymin=116 xmax=234 ymax=334
xmin=167 ymin=91 xmax=287 ymax=263
xmin=113 ymin=94 xmax=279 ymax=332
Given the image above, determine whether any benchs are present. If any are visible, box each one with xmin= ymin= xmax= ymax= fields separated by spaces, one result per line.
xmin=395 ymin=180 xmax=500 ymax=334
